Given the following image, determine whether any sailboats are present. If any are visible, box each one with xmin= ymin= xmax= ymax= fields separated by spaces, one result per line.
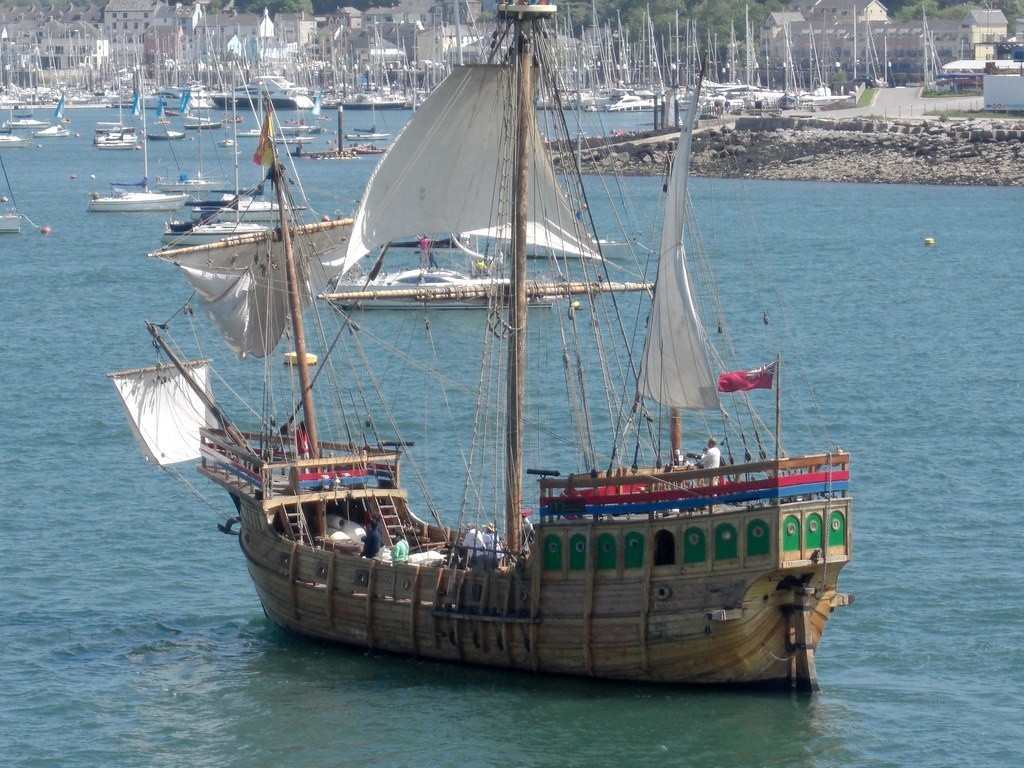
xmin=148 ymin=1 xmax=851 ymax=699
xmin=0 ymin=1 xmax=943 ymax=309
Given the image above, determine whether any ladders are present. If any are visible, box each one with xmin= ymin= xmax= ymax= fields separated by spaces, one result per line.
xmin=370 ymin=493 xmax=408 ymax=547
xmin=279 ymin=501 xmax=314 ymax=548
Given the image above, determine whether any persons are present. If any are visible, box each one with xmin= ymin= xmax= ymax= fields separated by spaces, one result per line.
xmin=479 ymin=523 xmax=505 ymax=570
xmin=390 ymin=530 xmax=410 ymax=601
xmin=415 ymin=233 xmax=439 ymax=269
xmin=700 ymin=437 xmax=721 ymax=513
xmin=476 ymin=259 xmax=488 ymax=273
xmin=361 ymin=513 xmax=385 ymax=562
xmin=287 ymin=416 xmax=311 ymax=490
xmin=577 ymin=202 xmax=587 ymax=225
xmin=462 ymin=522 xmax=488 ymax=570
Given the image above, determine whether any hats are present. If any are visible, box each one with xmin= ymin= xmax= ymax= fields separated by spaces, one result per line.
xmin=522 ymin=509 xmax=533 ymax=516
xmin=481 ymin=521 xmax=495 ymax=532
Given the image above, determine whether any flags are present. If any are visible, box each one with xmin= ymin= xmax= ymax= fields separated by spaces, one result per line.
xmin=718 ymin=361 xmax=775 ymax=393
xmin=312 ymin=92 xmax=322 ymax=115
xmin=252 ymin=99 xmax=278 ymax=166
xmin=178 ymin=87 xmax=191 ymax=114
xmin=131 ymin=90 xmax=141 ymax=116
xmin=53 ymin=95 xmax=64 ymax=118
xmin=156 ymin=98 xmax=165 ymax=120
